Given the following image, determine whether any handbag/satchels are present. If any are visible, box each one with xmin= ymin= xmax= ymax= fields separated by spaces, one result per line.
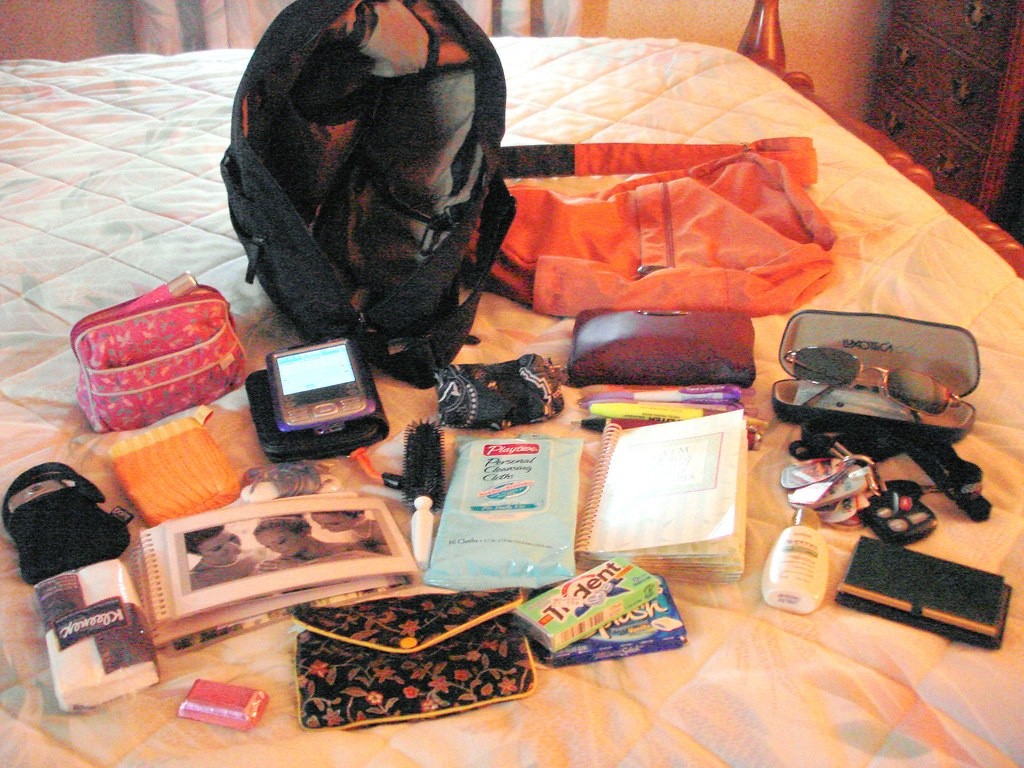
xmin=466 ymin=136 xmax=838 ymax=317
xmin=1 ymin=462 xmax=133 ymax=587
xmin=69 ymin=275 xmax=250 ymax=434
xmin=295 ymin=587 xmax=539 ymax=732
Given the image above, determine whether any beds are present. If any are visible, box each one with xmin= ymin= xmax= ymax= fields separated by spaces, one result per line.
xmin=0 ymin=0 xmax=1024 ymax=768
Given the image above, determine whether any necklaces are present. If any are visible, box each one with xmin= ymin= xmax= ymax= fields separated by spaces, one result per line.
xmin=199 ymin=554 xmax=241 ymax=568
xmin=354 ymin=519 xmax=373 ymax=538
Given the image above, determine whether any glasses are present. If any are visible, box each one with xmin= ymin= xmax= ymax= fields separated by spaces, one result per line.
xmin=784 ymin=346 xmax=962 ymax=438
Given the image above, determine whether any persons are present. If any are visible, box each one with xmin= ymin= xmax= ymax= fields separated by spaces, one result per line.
xmin=311 ymin=510 xmax=391 ymax=556
xmin=184 ymin=525 xmax=278 ymax=590
xmin=249 ymin=513 xmax=354 ymax=575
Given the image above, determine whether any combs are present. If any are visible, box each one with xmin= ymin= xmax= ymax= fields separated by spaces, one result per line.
xmin=400 ymin=418 xmax=446 ymax=568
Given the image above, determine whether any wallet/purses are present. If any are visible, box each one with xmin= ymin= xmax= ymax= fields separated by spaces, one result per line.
xmin=244 ymin=354 xmax=390 ymax=463
xmin=566 ymin=309 xmax=755 ymax=386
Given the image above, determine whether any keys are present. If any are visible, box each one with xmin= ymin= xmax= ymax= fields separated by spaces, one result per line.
xmin=880 ymin=480 xmax=942 ymax=500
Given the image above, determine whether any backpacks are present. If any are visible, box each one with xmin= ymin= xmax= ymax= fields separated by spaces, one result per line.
xmin=220 ymin=2 xmax=517 ymax=390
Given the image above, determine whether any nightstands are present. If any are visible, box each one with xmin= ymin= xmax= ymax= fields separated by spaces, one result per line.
xmin=871 ymin=0 xmax=1024 ymax=245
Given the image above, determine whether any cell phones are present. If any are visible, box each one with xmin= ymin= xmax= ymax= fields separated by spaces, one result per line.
xmin=266 ymin=337 xmax=376 ymax=433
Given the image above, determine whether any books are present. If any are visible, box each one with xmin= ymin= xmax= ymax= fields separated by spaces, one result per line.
xmin=575 ymin=409 xmax=748 ymax=581
xmin=132 ymin=489 xmax=419 ymax=649
